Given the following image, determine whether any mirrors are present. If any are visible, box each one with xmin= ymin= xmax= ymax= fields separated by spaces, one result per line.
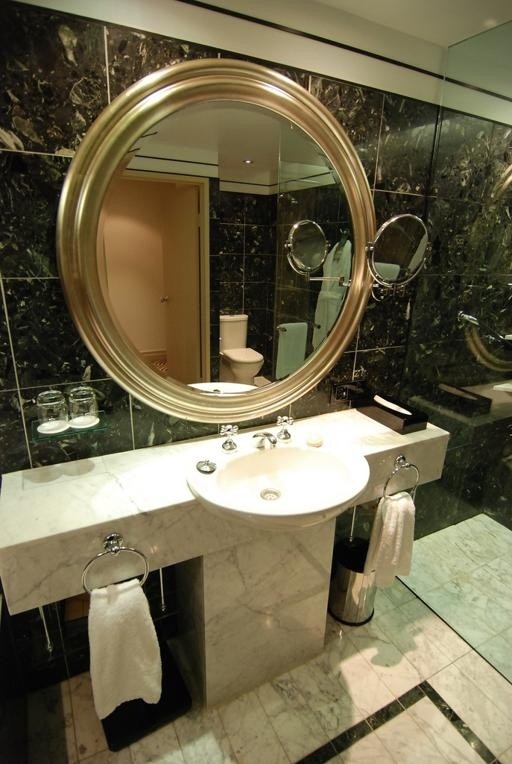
xmin=460 ymin=165 xmax=512 ymax=369
xmin=284 ymin=217 xmax=350 ymax=288
xmin=56 ymin=57 xmax=377 ymax=423
xmin=368 ymin=211 xmax=432 ymax=304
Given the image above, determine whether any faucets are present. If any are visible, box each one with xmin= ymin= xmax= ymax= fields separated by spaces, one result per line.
xmin=251 ymin=431 xmax=277 ymax=449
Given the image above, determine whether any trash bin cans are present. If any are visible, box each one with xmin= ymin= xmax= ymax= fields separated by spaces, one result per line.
xmin=327 ymin=537 xmax=376 ymax=626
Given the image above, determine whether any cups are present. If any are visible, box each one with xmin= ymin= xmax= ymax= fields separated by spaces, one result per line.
xmin=35 ymin=386 xmax=99 ymax=432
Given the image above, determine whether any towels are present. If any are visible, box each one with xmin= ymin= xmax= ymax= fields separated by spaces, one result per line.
xmin=374 ymin=262 xmax=400 ymax=281
xmin=364 ymin=492 xmax=414 ymax=588
xmin=275 ymin=321 xmax=308 ymax=381
xmin=88 ymin=578 xmax=162 ymax=720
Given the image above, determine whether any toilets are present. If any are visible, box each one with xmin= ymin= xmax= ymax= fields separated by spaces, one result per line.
xmin=219 ymin=314 xmax=265 ymax=387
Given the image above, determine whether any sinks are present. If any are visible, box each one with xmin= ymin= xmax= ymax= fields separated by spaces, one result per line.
xmin=188 ymin=383 xmax=259 ymax=395
xmin=306 ymin=435 xmax=321 ymax=446
xmin=187 ymin=439 xmax=372 ymax=529
